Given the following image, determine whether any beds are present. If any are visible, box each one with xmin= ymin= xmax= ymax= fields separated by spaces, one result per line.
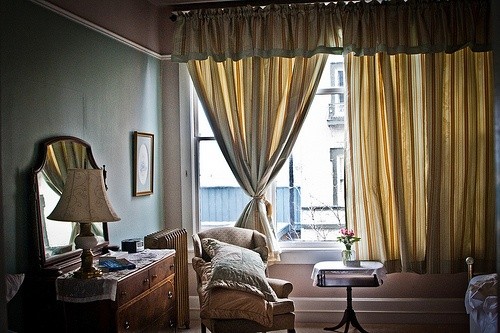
xmin=465 ymin=257 xmax=500 ymax=333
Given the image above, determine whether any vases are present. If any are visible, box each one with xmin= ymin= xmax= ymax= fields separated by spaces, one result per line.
xmin=342 ymin=250 xmax=355 ymax=265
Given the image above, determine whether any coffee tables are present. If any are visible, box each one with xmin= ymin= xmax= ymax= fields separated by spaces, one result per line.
xmin=310 ymin=260 xmax=387 ymax=333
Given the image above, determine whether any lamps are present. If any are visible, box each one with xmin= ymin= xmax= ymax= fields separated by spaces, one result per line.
xmin=46 ymin=168 xmax=121 ymax=277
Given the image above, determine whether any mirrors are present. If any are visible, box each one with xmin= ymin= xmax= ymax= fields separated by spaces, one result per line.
xmin=32 ymin=135 xmax=110 ymax=268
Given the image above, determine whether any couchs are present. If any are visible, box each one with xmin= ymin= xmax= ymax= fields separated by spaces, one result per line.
xmin=192 ymin=227 xmax=297 ymax=333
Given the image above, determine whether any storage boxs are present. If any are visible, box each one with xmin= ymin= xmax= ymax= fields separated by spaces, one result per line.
xmin=346 ymin=260 xmax=361 ymax=267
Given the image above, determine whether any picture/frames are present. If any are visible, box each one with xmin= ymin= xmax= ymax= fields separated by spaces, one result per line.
xmin=131 ymin=131 xmax=154 ymax=197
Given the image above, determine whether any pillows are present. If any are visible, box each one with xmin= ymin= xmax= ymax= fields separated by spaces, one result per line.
xmin=193 ymin=258 xmax=274 ymax=328
xmin=202 ymin=238 xmax=277 ymax=302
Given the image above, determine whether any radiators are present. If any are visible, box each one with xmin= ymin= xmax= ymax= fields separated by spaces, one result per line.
xmin=145 ymin=228 xmax=189 ymax=328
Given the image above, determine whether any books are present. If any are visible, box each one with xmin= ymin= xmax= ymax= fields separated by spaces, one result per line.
xmin=100 ymin=258 xmax=136 ymax=272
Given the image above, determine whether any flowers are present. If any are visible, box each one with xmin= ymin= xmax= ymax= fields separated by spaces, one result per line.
xmin=336 ymin=229 xmax=361 ymax=251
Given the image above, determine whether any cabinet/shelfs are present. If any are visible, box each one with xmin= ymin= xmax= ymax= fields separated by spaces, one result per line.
xmin=52 ymin=248 xmax=176 ymax=333
